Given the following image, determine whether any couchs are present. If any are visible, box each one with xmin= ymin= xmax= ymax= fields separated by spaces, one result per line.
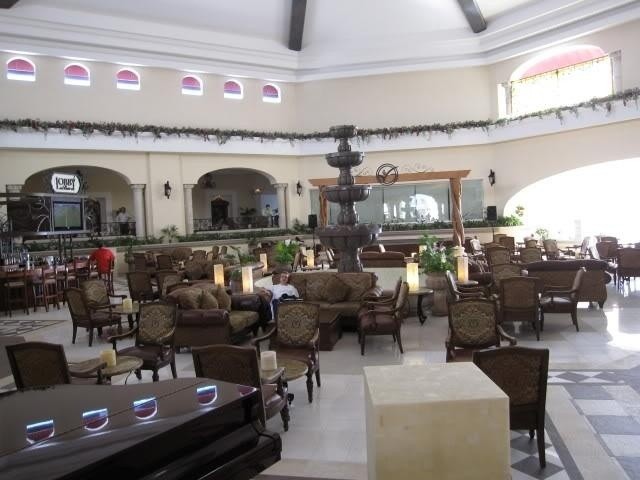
xmin=257 ymin=272 xmax=384 ymax=332
xmin=166 ymin=282 xmax=261 ymax=353
xmin=359 ymin=251 xmax=415 ymax=267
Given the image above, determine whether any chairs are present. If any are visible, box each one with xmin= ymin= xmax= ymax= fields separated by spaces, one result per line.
xmin=180 ymin=246 xmax=227 ymax=282
xmin=444 ymin=298 xmax=517 ymax=363
xmin=492 ymin=276 xmax=542 ymax=341
xmin=107 ymin=300 xmax=180 ymax=382
xmin=357 ymin=277 xmax=409 ymax=356
xmin=251 ymin=299 xmax=321 ymax=404
xmin=125 ymin=245 xmax=179 ymax=300
xmin=0 ymin=252 xmax=133 ymax=347
xmin=470 ymin=346 xmax=551 ymax=469
xmin=191 ymin=344 xmax=289 ymax=432
xmin=445 ymin=233 xmax=639 ymax=310
xmin=5 ymin=341 xmax=107 ymax=393
xmin=541 ymin=266 xmax=586 ymax=332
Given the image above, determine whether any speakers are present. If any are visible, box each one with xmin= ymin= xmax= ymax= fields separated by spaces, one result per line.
xmin=308 ymin=214 xmax=317 ymax=229
xmin=487 ymin=206 xmax=497 ymax=221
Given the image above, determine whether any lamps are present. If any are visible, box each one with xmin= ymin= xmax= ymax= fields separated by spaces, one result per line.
xmin=407 ymin=262 xmax=419 ymax=291
xmin=260 ymin=254 xmax=267 ymax=271
xmin=307 ymin=249 xmax=314 ymax=268
xmin=242 ymin=266 xmax=253 ymax=293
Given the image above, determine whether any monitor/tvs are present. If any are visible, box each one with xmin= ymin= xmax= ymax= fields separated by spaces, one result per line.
xmin=53 ymin=200 xmax=83 ymax=231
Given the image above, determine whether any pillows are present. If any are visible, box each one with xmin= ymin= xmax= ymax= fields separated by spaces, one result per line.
xmin=218 ymin=284 xmax=231 ymax=313
xmin=201 ymin=289 xmax=219 ymax=309
xmin=321 ymin=275 xmax=351 ymax=304
xmin=306 ymin=276 xmax=330 ymax=298
xmin=287 ymin=277 xmax=306 ymax=300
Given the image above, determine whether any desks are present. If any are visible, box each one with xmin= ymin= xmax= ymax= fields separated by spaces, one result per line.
xmin=101 ymin=355 xmax=144 ymax=384
xmin=408 ymin=287 xmax=434 ymax=325
xmin=361 ymin=361 xmax=511 ymax=480
xmin=257 ymin=358 xmax=308 ymax=381
xmin=0 ymin=380 xmax=283 ymax=479
xmin=319 ymin=311 xmax=342 ymax=350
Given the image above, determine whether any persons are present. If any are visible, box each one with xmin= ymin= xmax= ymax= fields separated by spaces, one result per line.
xmin=87 ymin=242 xmax=115 ymax=279
xmin=112 ymin=206 xmax=132 ymax=237
xmin=206 ymin=245 xmax=229 ymax=263
xmin=262 ymin=203 xmax=273 ymax=217
xmin=266 ymin=270 xmax=299 ymax=322
